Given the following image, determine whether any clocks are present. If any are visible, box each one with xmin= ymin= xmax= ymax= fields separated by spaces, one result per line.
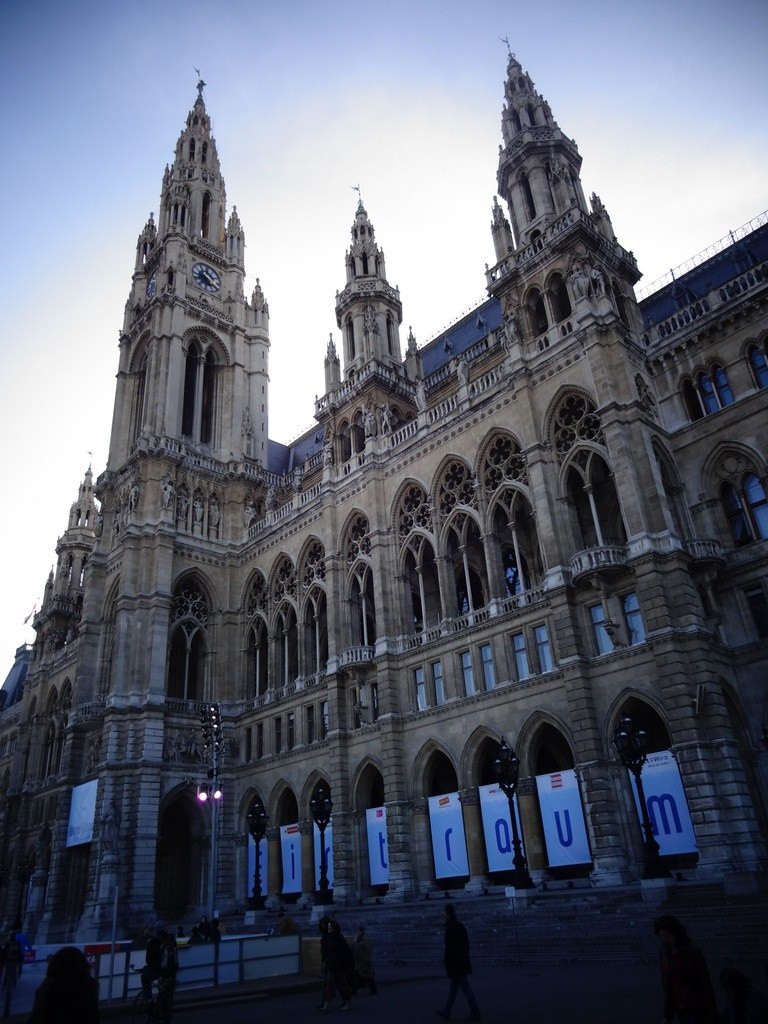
xmin=192 ymin=263 xmax=221 ymax=293
xmin=146 ymin=269 xmax=156 ymax=297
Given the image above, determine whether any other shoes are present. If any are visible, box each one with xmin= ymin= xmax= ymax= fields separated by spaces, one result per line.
xmin=320 ymin=1002 xmax=332 ymax=1011
xmin=436 ymin=1008 xmax=450 ymax=1020
xmin=464 ymin=1014 xmax=481 ymax=1022
xmin=339 ymin=1000 xmax=353 ymax=1010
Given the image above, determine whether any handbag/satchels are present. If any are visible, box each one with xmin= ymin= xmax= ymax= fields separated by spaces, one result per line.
xmin=336 ymin=946 xmax=354 ymax=969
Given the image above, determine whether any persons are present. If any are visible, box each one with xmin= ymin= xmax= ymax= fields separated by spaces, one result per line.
xmin=245 ymin=500 xmax=257 ymax=526
xmin=163 ymin=481 xmax=175 ymax=506
xmin=317 ymin=916 xmax=353 ymax=1012
xmin=187 ymin=915 xmax=225 ymax=944
xmin=130 ymin=484 xmax=139 ymax=511
xmin=28 ymin=946 xmax=100 ymax=1024
xmin=0 ymin=931 xmax=33 ymax=993
xmin=570 ymin=264 xmax=589 ymax=297
xmin=278 ymin=912 xmax=297 ymax=935
xmin=353 ymin=927 xmax=374 ymax=1001
xmin=135 ymin=914 xmax=179 ymax=1024
xmin=414 ymin=380 xmax=428 ymax=410
xmin=653 ymin=914 xmax=718 ymax=1024
xmin=177 ymin=490 xmax=220 ymax=526
xmin=434 ymin=904 xmax=480 ymax=1024
xmin=380 ymin=404 xmax=394 ymax=435
xmin=265 ymin=485 xmax=275 ymax=511
xmin=291 ymin=469 xmax=303 ymax=492
xmin=361 ymin=409 xmax=374 ymax=437
xmin=320 ymin=439 xmax=334 ymax=467
xmin=176 ymin=926 xmax=186 ymax=938
xmin=591 ymin=264 xmax=605 ymax=294
xmin=716 ymin=962 xmax=768 ymax=1024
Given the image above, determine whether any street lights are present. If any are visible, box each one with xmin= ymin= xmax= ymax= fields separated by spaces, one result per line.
xmin=493 ymin=735 xmax=540 ymax=898
xmin=14 ymin=856 xmax=37 ymax=929
xmin=197 ymin=703 xmax=225 ymax=932
xmin=309 ymin=787 xmax=338 ymax=911
xmin=246 ymin=803 xmax=269 ymax=916
xmin=618 ymin=716 xmax=661 ymax=856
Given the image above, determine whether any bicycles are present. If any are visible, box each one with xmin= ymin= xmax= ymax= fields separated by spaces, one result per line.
xmin=130 ymin=964 xmax=174 ymax=1024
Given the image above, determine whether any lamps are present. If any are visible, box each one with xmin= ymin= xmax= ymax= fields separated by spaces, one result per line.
xmin=214 ymin=729 xmax=223 ymax=736
xmin=213 ymin=724 xmax=220 ymax=728
xmin=214 ymin=783 xmax=224 ymax=799
xmin=210 ymin=703 xmax=219 ymax=712
xmin=203 ymin=740 xmax=212 ymax=749
xmin=214 ymin=737 xmax=224 ymax=745
xmin=212 ymin=713 xmax=219 ymax=719
xmin=201 ymin=725 xmax=213 ymax=732
xmin=197 ymin=783 xmax=209 ymax=802
